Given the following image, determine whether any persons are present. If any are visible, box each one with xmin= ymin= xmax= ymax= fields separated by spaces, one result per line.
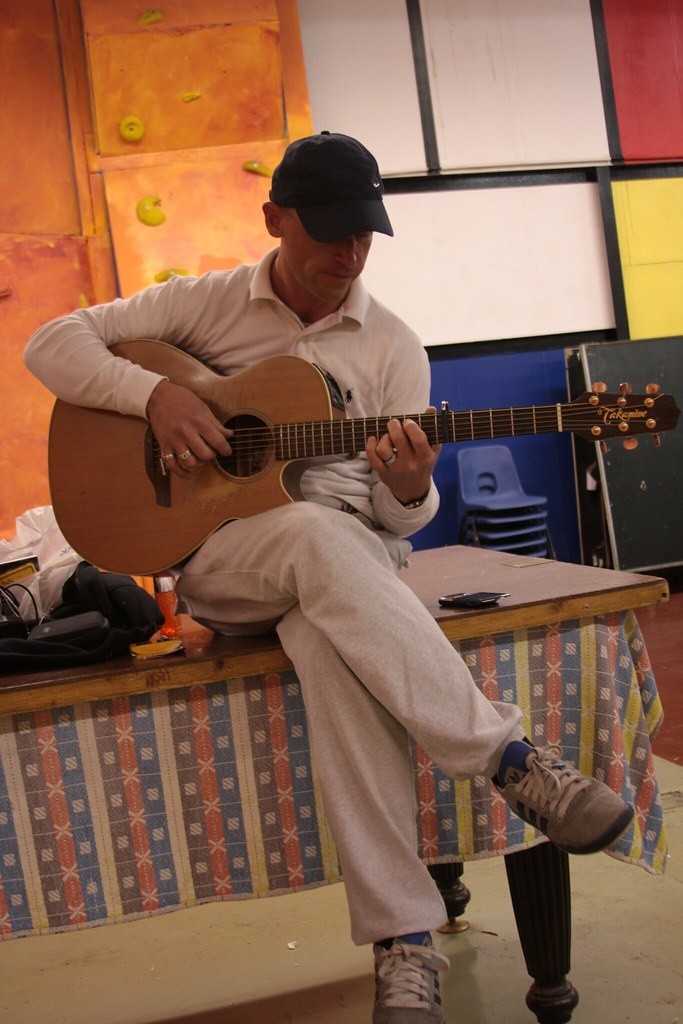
xmin=22 ymin=129 xmax=635 ymax=1022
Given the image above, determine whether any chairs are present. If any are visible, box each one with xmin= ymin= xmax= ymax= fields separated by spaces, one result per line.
xmin=457 ymin=445 xmax=555 ymax=561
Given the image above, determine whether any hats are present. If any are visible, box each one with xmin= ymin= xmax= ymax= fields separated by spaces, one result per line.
xmin=272 ymin=131 xmax=394 ymax=243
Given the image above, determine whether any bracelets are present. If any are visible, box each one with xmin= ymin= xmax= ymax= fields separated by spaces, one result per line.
xmin=404 ymin=498 xmax=426 ymax=510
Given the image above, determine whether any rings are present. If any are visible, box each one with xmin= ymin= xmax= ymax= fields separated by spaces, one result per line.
xmin=179 ymin=451 xmax=191 ymax=460
xmin=162 ymin=453 xmax=175 ymax=461
xmin=385 ymin=454 xmax=397 ymax=465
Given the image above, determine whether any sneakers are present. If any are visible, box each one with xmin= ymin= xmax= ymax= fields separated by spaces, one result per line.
xmin=373 ymin=931 xmax=451 ymax=1024
xmin=493 ymin=736 xmax=634 ymax=855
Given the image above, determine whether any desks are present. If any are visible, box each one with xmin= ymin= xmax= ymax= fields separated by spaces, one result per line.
xmin=0 ymin=543 xmax=670 ymax=1024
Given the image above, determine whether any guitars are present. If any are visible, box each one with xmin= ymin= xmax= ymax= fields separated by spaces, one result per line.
xmin=42 ymin=333 xmax=683 ymax=584
